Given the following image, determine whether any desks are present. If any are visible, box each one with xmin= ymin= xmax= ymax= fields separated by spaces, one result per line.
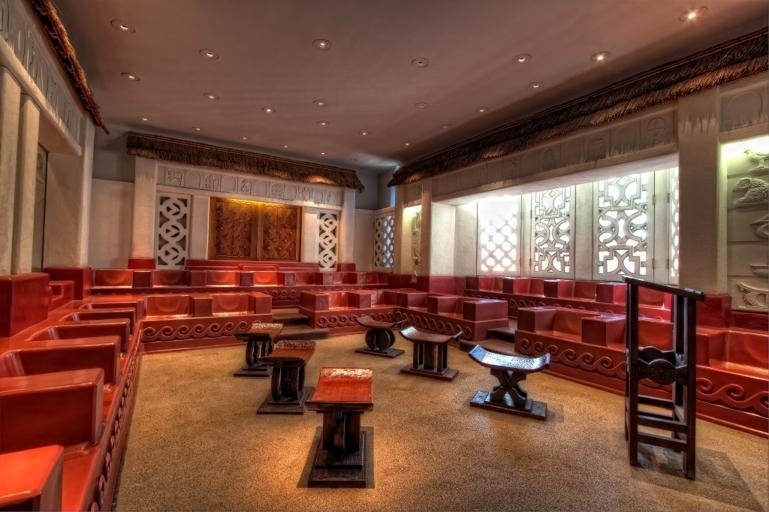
xmin=304 ymin=366 xmax=374 ymax=488
xmin=233 ymin=322 xmax=284 ymax=377
xmin=399 ymin=326 xmax=463 ymax=382
xmin=354 ymin=315 xmax=407 ymax=361
xmin=256 ymin=339 xmax=317 ymax=415
xmin=468 ymin=344 xmax=551 ymax=421
